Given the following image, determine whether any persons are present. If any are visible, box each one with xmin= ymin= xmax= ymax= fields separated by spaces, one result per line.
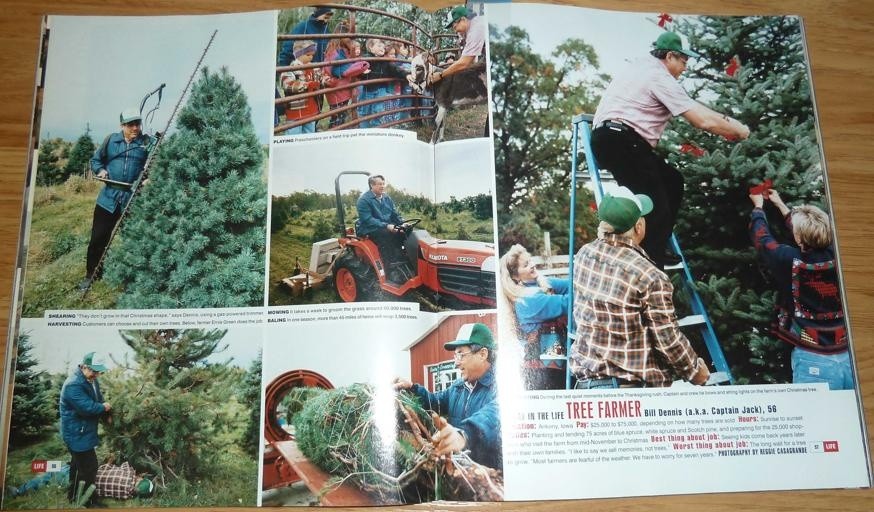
xmin=93 ymin=456 xmax=156 ymax=500
xmin=748 ymin=187 xmax=856 ymax=390
xmin=275 ymin=6 xmax=486 ymax=135
xmin=393 ymin=323 xmax=502 ymax=472
xmin=567 ymin=185 xmax=711 ymax=388
xmin=77 ymin=110 xmax=158 ymax=290
xmin=500 ymin=244 xmax=575 ymax=390
xmin=592 ymin=28 xmax=751 ymax=262
xmin=357 ymin=174 xmax=413 ymax=278
xmin=8 ymin=458 xmax=70 ymax=496
xmin=60 ymin=353 xmax=114 ymax=508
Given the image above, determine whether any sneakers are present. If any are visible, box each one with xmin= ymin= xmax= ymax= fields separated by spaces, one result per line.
xmin=78 ymin=278 xmax=92 ymax=289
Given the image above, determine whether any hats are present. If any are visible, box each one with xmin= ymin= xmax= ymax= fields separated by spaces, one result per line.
xmin=82 ymin=352 xmax=109 ymax=372
xmin=443 ymin=322 xmax=496 ymax=351
xmin=444 ymin=7 xmax=468 ymax=28
xmin=313 ymin=7 xmax=330 ymax=18
xmin=292 ymin=39 xmax=317 ymax=59
xmin=598 ymin=186 xmax=654 ymax=234
xmin=120 ymin=109 xmax=142 ymax=125
xmin=655 ymin=31 xmax=701 ymax=59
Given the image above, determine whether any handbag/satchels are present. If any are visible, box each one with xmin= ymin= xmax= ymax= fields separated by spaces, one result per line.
xmin=94 ymin=450 xmax=135 ymax=499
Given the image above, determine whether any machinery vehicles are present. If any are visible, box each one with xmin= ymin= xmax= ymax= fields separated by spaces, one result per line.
xmin=282 ymin=167 xmax=498 ymax=308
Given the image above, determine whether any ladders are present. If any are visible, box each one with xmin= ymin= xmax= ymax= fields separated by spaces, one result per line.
xmin=565 ymin=114 xmax=735 ymax=390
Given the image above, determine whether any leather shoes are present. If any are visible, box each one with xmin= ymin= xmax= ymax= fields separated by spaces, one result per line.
xmin=661 ymin=240 xmax=683 ymax=265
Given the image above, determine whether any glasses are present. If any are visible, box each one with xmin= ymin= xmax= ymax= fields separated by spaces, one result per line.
xmin=453 ymin=351 xmax=472 ymax=360
xmin=326 ymin=12 xmax=333 ymax=16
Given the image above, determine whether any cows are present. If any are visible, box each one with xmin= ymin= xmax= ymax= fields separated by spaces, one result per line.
xmin=409 ymin=51 xmax=488 ymax=144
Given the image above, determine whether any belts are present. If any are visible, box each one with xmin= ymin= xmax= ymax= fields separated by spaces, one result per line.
xmin=576 ymin=378 xmax=630 ymax=389
xmin=590 ymin=121 xmax=629 ymax=132
